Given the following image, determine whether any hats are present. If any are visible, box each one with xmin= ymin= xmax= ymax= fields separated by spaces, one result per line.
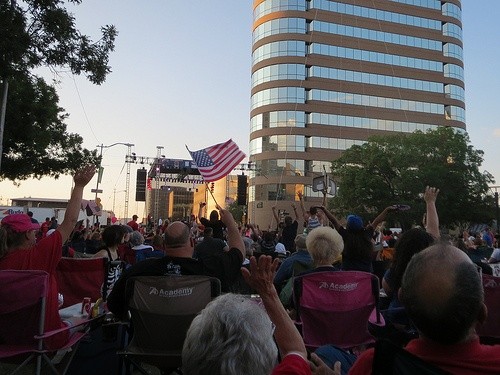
xmin=2 ymin=214 xmax=40 ymax=231
xmin=348 ymin=214 xmax=362 ymax=227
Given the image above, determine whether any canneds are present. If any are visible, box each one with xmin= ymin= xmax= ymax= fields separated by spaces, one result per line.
xmin=81 ymin=297 xmax=91 ymax=314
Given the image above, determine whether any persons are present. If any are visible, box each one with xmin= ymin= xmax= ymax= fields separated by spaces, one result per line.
xmin=0 ymin=162 xmax=500 ymax=375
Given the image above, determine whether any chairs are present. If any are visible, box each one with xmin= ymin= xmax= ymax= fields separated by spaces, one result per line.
xmin=0 ymin=236 xmax=500 ymax=375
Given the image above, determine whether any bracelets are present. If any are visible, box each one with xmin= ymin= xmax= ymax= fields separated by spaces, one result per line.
xmin=294 ymin=208 xmax=296 ymax=210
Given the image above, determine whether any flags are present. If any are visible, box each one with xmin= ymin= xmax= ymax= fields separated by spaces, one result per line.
xmin=190 ymin=139 xmax=246 ymax=183
